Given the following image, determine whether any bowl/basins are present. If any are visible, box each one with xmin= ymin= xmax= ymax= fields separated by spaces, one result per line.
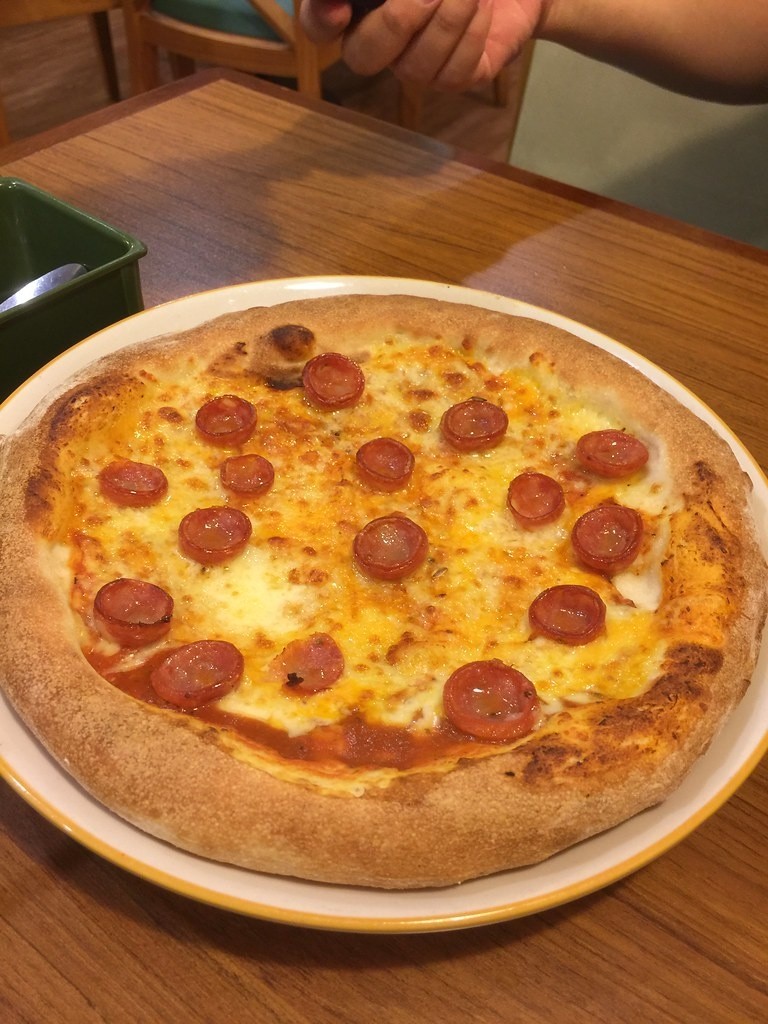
xmin=0 ymin=176 xmax=148 ymax=399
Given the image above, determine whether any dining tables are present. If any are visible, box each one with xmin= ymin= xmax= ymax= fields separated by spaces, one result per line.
xmin=0 ymin=68 xmax=768 ymax=1024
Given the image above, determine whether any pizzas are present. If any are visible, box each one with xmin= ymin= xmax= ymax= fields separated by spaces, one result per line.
xmin=0 ymin=293 xmax=766 ymax=887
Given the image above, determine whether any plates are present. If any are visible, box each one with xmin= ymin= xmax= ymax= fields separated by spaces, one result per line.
xmin=0 ymin=274 xmax=768 ymax=935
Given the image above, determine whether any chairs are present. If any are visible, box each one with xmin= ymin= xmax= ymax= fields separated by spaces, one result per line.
xmin=122 ymin=0 xmax=518 ymax=129
